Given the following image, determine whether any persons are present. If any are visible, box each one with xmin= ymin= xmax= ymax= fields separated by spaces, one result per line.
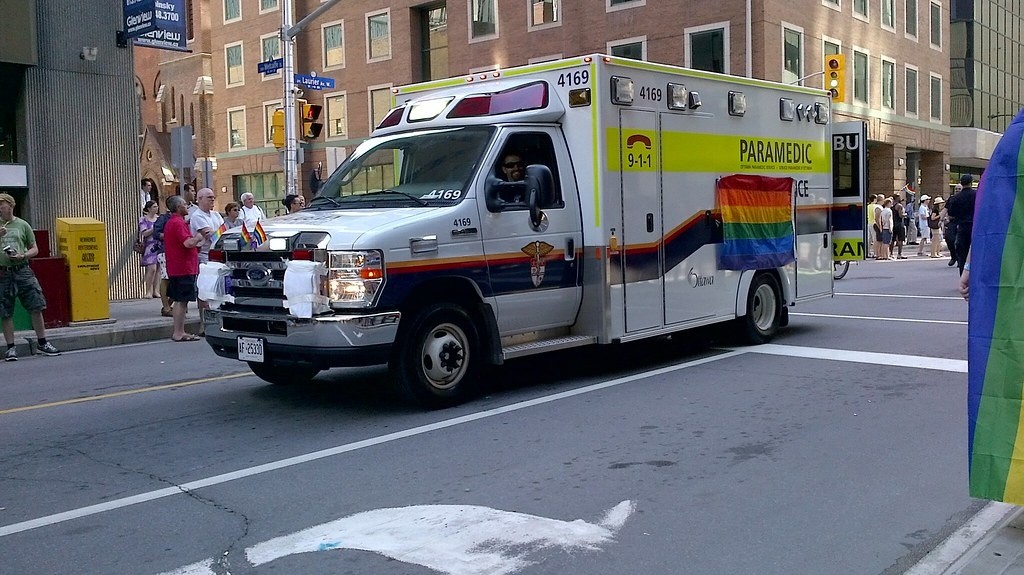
xmin=152 ymin=210 xmax=173 ymax=318
xmin=164 ymin=196 xmax=204 ymax=342
xmin=938 ymin=174 xmax=976 ymax=277
xmin=239 ymin=192 xmax=266 ymax=223
xmin=184 ymin=183 xmax=198 ymax=220
xmin=141 ymin=178 xmax=152 ymax=204
xmin=499 ymin=150 xmax=526 ymax=203
xmin=139 ymin=200 xmax=162 ymax=299
xmin=959 ymin=245 xmax=971 ymax=301
xmin=0 ymin=193 xmax=60 ymax=361
xmin=224 ymin=203 xmax=243 ymax=229
xmin=191 ymin=188 xmax=224 ymax=336
xmin=867 ymin=194 xmax=945 ymax=260
xmin=281 ymin=195 xmax=305 ymax=212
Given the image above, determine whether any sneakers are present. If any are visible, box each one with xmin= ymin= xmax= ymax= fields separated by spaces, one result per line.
xmin=4 ymin=345 xmax=18 ymax=362
xmin=36 ymin=342 xmax=61 ymax=355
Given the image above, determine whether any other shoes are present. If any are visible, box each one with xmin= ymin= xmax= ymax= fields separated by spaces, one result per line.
xmin=161 ymin=306 xmax=174 ymax=316
xmin=146 ymin=293 xmax=154 ymax=299
xmin=867 ymin=239 xmax=958 ymax=266
xmin=153 ymin=294 xmax=161 ymax=297
xmin=198 ymin=330 xmax=205 ymax=337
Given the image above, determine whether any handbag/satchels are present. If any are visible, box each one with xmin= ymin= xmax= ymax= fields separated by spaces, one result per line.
xmin=133 ymin=242 xmax=145 ymax=254
xmin=927 ymin=209 xmax=939 ymax=229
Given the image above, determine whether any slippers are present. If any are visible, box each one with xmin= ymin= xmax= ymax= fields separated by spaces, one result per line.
xmin=172 ymin=334 xmax=200 ymax=342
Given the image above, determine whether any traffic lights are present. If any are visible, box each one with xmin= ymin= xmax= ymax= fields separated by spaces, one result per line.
xmin=301 ymin=103 xmax=323 ymax=141
xmin=825 ymin=54 xmax=845 ymax=102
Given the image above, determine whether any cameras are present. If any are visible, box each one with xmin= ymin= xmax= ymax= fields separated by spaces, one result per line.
xmin=3 ymin=245 xmax=17 ymax=257
xmin=150 ymin=243 xmax=162 ymax=253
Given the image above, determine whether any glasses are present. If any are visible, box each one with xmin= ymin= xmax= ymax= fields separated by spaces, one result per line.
xmin=151 ymin=205 xmax=159 ymax=209
xmin=294 ymin=201 xmax=303 ymax=206
xmin=504 ymin=161 xmax=524 ymax=169
xmin=202 ymin=195 xmax=216 ymax=200
xmin=231 ymin=209 xmax=240 ymax=212
xmin=146 ymin=185 xmax=151 ymax=188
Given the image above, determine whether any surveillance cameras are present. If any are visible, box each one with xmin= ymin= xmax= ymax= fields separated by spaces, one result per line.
xmin=293 ymin=87 xmax=303 ymax=97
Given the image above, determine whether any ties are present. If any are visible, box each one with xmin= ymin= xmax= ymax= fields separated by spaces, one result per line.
xmin=145 ymin=192 xmax=147 ymax=203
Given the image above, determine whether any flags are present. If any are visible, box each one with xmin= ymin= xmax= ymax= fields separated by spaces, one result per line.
xmin=212 ymin=225 xmax=227 ymax=241
xmin=240 ymin=222 xmax=267 ymax=245
xmin=717 ymin=173 xmax=795 ymax=271
xmin=966 ymin=107 xmax=1024 ymax=506
xmin=904 ymin=185 xmax=916 ymax=195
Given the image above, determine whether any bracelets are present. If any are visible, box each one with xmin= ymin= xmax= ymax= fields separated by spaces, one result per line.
xmin=964 ymin=263 xmax=970 ymax=271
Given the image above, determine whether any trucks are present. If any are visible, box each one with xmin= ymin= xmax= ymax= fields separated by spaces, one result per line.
xmin=196 ymin=53 xmax=869 ymax=411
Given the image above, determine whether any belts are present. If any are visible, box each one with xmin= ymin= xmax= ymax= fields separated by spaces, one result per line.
xmin=911 ymin=218 xmax=914 ymax=219
xmin=0 ymin=263 xmax=29 ymax=272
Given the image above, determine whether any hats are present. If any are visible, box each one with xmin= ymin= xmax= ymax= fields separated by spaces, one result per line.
xmin=0 ymin=193 xmax=16 ymax=207
xmin=920 ymin=195 xmax=932 ymax=201
xmin=934 ymin=196 xmax=945 ymax=204
